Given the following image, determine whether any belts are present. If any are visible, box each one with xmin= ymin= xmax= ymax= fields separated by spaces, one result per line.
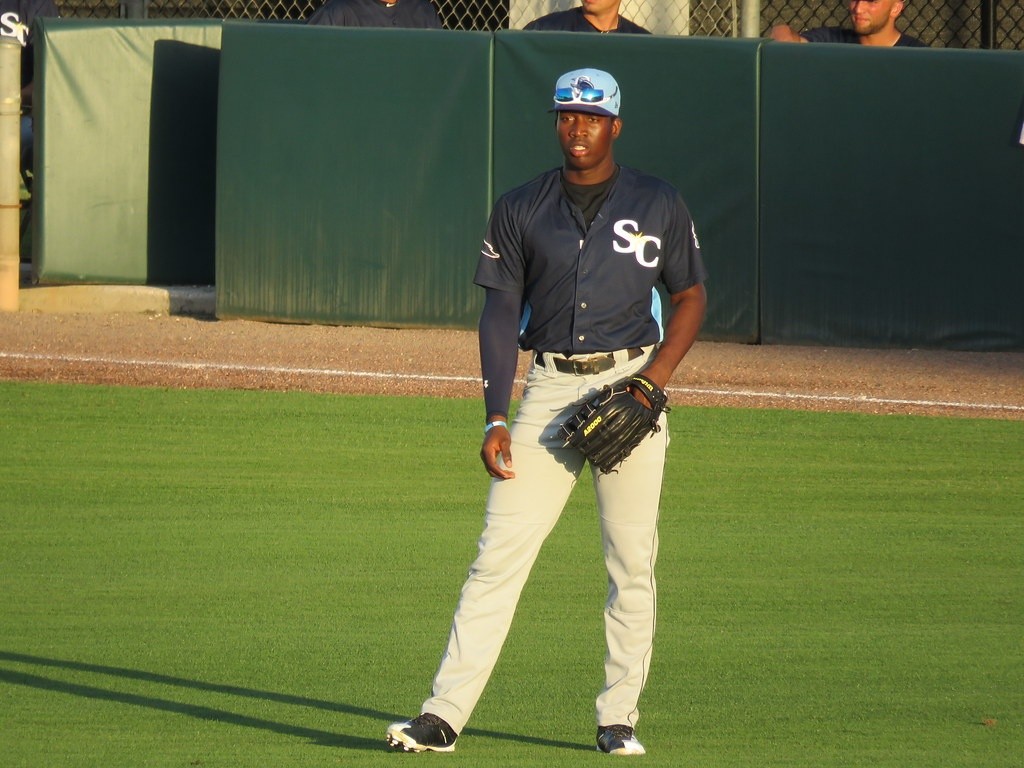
xmin=536 ymin=346 xmax=643 ymax=376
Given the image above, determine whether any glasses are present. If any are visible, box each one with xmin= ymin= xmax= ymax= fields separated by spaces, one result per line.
xmin=552 ymin=87 xmax=618 ymax=104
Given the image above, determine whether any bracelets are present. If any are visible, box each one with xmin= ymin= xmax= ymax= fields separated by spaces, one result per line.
xmin=484 ymin=421 xmax=507 ymax=435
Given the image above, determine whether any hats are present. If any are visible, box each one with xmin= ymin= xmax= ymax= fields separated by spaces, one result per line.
xmin=547 ymin=67 xmax=620 ymax=117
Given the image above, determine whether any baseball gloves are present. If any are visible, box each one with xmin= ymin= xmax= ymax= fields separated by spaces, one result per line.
xmin=559 ymin=373 xmax=668 ymax=475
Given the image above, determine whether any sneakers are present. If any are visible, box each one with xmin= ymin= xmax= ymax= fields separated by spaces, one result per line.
xmin=386 ymin=712 xmax=459 ymax=753
xmin=595 ymin=725 xmax=646 ymax=755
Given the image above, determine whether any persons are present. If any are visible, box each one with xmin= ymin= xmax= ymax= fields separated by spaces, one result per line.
xmin=769 ymin=0 xmax=928 ymax=48
xmin=522 ymin=0 xmax=652 ymax=35
xmin=0 ymin=0 xmax=58 ymax=170
xmin=308 ymin=0 xmax=443 ymax=29
xmin=387 ymin=69 xmax=710 ymax=756
